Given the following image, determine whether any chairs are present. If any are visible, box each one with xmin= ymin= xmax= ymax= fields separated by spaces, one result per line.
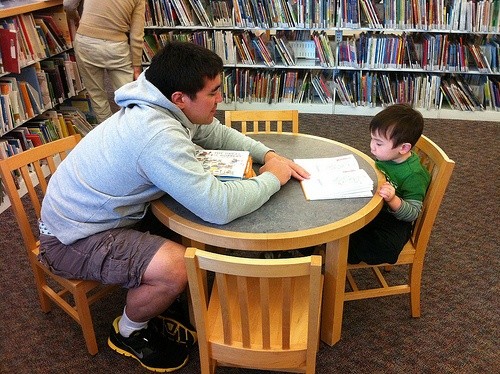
xmin=0 ymin=136 xmax=135 ymax=356
xmin=183 ymin=248 xmax=326 ymax=374
xmin=321 ymin=133 xmax=455 ymax=317
xmin=225 ymin=109 xmax=300 ymax=135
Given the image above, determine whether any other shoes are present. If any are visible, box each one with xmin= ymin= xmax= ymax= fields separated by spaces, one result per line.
xmin=255 ymin=251 xmax=287 ymax=259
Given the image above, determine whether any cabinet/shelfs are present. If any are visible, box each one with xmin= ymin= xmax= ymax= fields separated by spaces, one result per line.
xmin=0 ymin=0 xmax=98 ymax=213
xmin=150 ymin=0 xmax=500 ymax=124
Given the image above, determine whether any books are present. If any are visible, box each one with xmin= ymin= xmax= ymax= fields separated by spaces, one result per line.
xmin=293 ymin=152 xmax=374 ymax=201
xmin=142 ymin=0 xmax=500 ymax=112
xmin=0 ymin=12 xmax=97 ymax=206
xmin=195 ymin=148 xmax=257 ymax=182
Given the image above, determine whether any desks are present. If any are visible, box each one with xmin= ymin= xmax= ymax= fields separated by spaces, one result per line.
xmin=153 ymin=133 xmax=384 ymax=347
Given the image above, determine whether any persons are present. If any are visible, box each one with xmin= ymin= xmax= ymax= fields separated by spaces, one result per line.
xmin=258 ymin=104 xmax=430 ymax=266
xmin=63 ymin=0 xmax=146 ymax=124
xmin=38 ymin=42 xmax=313 ymax=374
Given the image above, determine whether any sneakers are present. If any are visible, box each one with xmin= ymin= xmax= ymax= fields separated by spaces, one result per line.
xmin=108 ymin=315 xmax=189 ymax=372
xmin=151 ymin=296 xmax=198 ymax=347
